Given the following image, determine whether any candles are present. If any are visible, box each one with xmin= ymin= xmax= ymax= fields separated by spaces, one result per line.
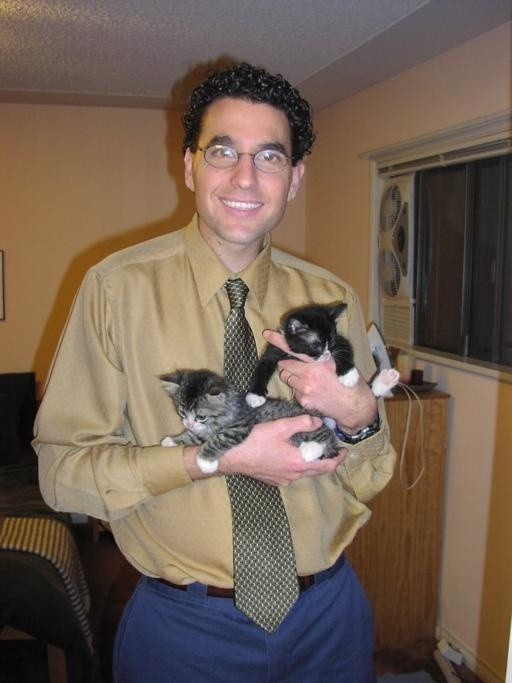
xmin=410 ymin=366 xmax=424 ymax=386
xmin=395 ymin=351 xmax=416 ymax=385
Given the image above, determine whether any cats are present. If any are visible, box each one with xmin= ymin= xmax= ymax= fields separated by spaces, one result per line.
xmin=156 ymin=368 xmax=340 ymax=476
xmin=244 ymin=300 xmax=403 ymax=431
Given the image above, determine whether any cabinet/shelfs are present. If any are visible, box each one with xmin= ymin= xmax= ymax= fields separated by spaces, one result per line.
xmin=341 ymin=380 xmax=451 ymax=674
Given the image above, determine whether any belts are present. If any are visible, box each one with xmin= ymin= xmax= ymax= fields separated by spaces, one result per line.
xmin=150 ymin=565 xmax=341 ymax=599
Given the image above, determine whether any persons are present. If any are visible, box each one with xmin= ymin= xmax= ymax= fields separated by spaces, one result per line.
xmin=30 ymin=61 xmax=397 ymax=683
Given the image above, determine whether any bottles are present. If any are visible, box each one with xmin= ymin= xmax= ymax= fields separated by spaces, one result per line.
xmin=390 ymin=347 xmax=424 ymax=385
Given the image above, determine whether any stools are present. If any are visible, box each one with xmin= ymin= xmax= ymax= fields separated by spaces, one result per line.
xmin=0 ymin=502 xmax=93 ymax=683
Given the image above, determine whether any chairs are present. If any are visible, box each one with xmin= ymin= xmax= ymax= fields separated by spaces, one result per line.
xmin=1 ymin=369 xmax=103 ymax=546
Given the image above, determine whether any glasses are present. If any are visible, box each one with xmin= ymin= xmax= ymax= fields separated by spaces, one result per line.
xmin=190 ymin=139 xmax=303 ymax=173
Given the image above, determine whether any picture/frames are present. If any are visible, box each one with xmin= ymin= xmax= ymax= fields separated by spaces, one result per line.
xmin=0 ymin=249 xmax=6 ymax=321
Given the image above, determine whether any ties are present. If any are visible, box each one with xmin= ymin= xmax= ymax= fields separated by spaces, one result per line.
xmin=220 ymin=276 xmax=303 ymax=634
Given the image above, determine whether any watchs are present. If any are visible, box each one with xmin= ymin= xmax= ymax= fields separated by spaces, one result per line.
xmin=334 ymin=418 xmax=382 ymax=444
